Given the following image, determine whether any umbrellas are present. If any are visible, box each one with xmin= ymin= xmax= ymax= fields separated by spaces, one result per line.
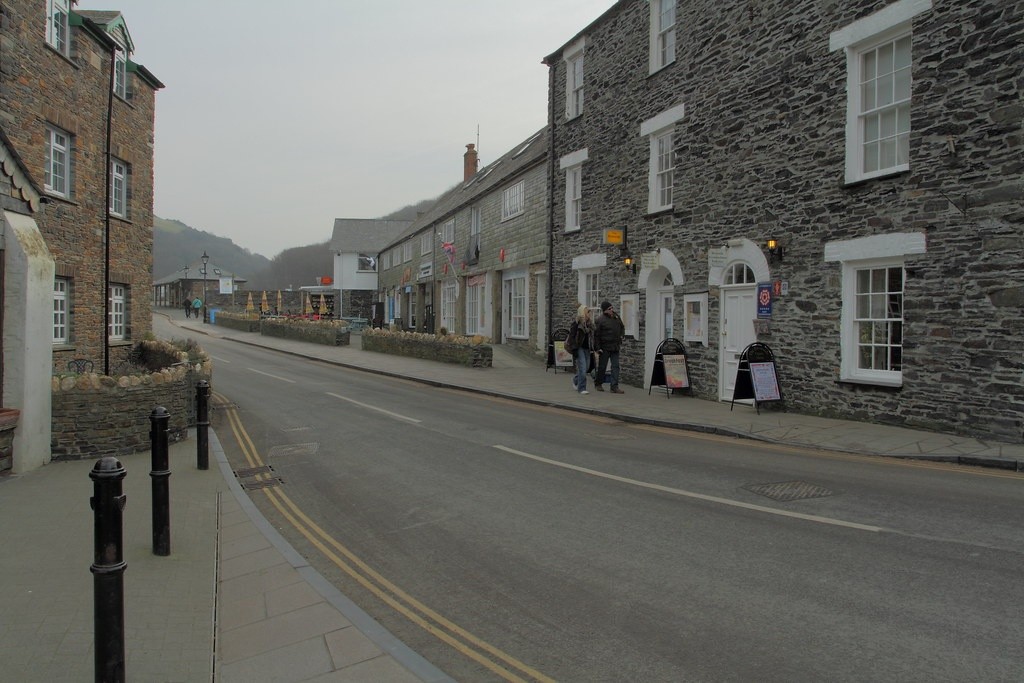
xmin=261 ymin=291 xmax=269 ymax=315
xmin=305 ymin=294 xmax=314 ymax=317
xmin=319 ymin=294 xmax=329 ymax=318
xmin=247 ymin=291 xmax=254 ymax=316
xmin=277 ymin=290 xmax=282 ymax=317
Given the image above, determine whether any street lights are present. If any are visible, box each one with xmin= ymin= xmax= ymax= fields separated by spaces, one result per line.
xmin=183 ymin=265 xmax=189 ymax=297
xmin=199 ymin=249 xmax=209 ymax=322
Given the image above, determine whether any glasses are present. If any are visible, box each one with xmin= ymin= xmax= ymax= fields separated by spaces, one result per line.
xmin=608 ymin=307 xmax=613 ymax=310
xmin=588 ymin=310 xmax=591 ymax=313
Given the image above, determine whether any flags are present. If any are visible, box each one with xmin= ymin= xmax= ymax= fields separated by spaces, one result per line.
xmin=441 ymin=242 xmax=455 ymax=264
xmin=361 ymin=256 xmax=376 ymax=272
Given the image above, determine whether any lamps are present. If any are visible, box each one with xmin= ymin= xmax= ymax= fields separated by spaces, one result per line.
xmin=625 ymin=256 xmax=636 ymax=274
xmin=765 ymin=234 xmax=782 ymax=259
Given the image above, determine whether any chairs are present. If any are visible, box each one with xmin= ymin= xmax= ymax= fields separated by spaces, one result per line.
xmin=68 ymin=359 xmax=94 ymax=374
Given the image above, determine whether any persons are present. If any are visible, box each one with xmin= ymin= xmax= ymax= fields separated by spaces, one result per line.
xmin=593 ymin=302 xmax=625 ymax=393
xmin=182 ymin=296 xmax=202 ymax=319
xmin=564 ymin=301 xmax=597 ymax=395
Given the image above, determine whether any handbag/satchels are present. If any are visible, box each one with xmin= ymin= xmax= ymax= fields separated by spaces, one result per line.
xmin=564 ymin=329 xmax=579 ymax=353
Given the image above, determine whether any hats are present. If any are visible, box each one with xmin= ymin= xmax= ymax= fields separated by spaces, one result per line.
xmin=601 ymin=301 xmax=612 ymax=311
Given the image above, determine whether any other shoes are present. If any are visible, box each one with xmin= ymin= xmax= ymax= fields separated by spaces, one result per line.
xmin=610 ymin=385 xmax=624 ymax=394
xmin=581 ymin=390 xmax=589 ymax=394
xmin=595 ymin=384 xmax=604 ymax=392
xmin=572 ymin=378 xmax=579 ymax=390
